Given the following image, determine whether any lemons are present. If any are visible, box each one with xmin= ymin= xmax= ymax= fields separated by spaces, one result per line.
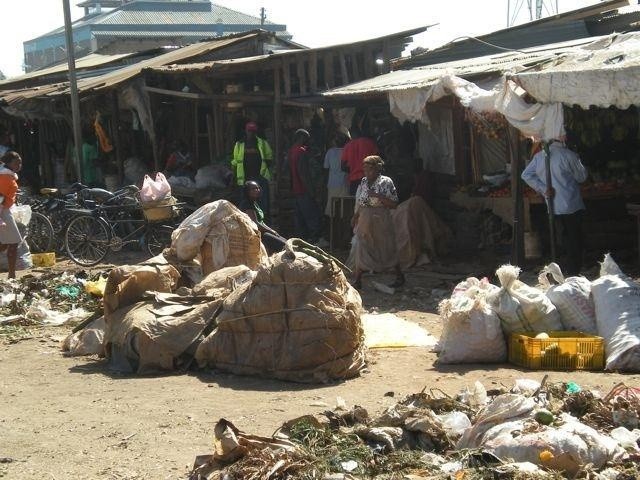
xmin=534 ymin=410 xmax=553 ymax=425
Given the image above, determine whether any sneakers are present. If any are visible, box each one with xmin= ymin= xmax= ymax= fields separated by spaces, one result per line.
xmin=394 ymin=274 xmax=405 ymax=286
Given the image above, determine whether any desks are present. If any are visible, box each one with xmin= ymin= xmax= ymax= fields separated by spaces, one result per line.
xmin=466 ymin=191 xmax=625 ymax=252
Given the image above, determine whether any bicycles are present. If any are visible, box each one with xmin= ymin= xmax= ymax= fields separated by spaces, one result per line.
xmin=16 ymin=182 xmax=186 ymax=266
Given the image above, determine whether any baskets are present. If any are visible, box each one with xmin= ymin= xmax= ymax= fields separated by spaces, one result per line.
xmin=510 ymin=330 xmax=604 ymax=370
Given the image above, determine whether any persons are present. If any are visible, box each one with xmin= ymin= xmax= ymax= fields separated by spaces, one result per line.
xmin=1 ymin=150 xmax=23 ymax=279
xmin=519 ymin=129 xmax=591 ymax=274
xmin=40 ymin=130 xmax=153 ymax=197
xmin=166 ymin=116 xmax=442 ymax=248
